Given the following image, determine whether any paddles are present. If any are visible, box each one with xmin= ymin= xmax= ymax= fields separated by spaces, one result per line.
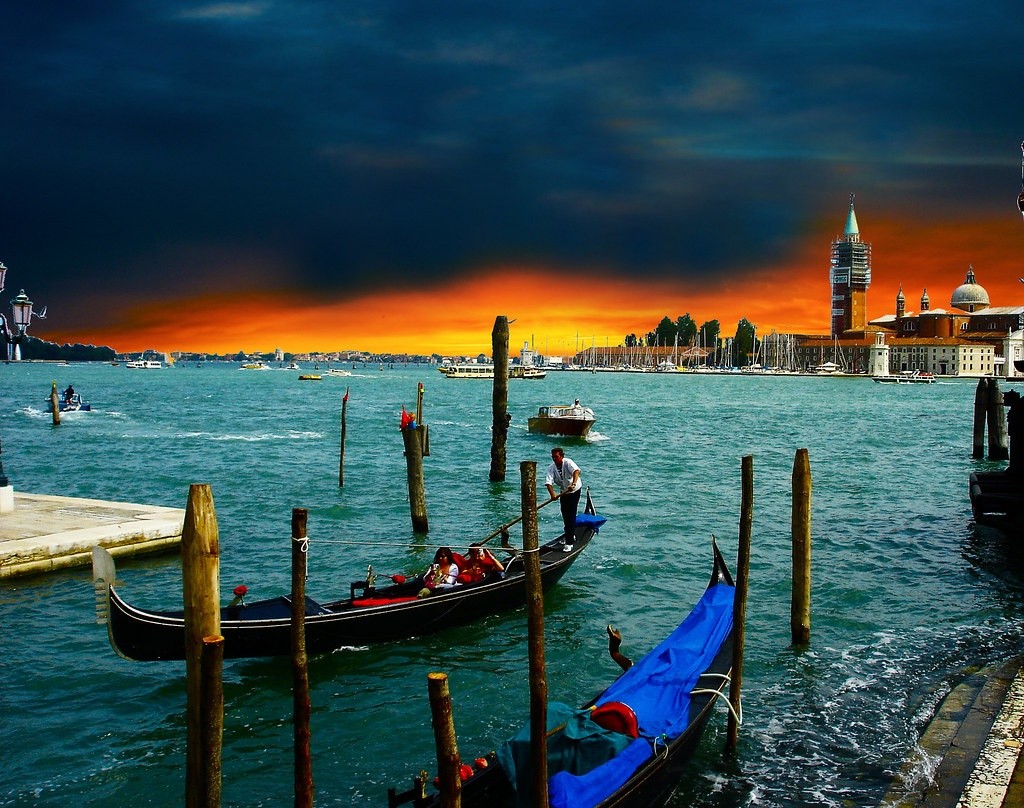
xmin=462 ymin=489 xmax=571 ymax=558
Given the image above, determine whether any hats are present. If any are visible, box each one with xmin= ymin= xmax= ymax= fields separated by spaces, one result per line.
xmin=575 ymin=398 xmax=580 ymax=401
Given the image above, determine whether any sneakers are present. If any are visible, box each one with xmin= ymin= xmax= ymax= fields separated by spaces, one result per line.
xmin=563 ymin=544 xmax=573 ymax=552
xmin=558 ymin=535 xmax=576 ymax=545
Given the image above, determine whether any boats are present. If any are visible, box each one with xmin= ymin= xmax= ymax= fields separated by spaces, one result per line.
xmin=298 ymin=374 xmax=322 ymax=380
xmin=286 ymin=362 xmax=299 ymax=370
xmin=528 ymin=406 xmax=597 ymax=439
xmin=871 ymin=369 xmax=935 ymax=384
xmin=386 ymin=535 xmax=737 ymax=808
xmin=126 ymin=360 xmax=162 ymax=369
xmin=44 ymin=389 xmax=91 ymax=413
xmin=325 ymin=369 xmax=351 ymax=376
xmin=92 ymin=485 xmax=608 ymax=664
xmin=238 ymin=361 xmax=271 ymax=370
xmin=446 ymin=365 xmax=547 ymax=380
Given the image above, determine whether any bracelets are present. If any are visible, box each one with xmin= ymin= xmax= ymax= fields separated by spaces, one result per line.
xmin=491 ymin=556 xmax=495 ymax=559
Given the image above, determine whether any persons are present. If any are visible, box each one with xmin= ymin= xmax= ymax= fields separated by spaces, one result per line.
xmin=459 ymin=543 xmax=504 ymax=583
xmin=419 ymin=547 xmax=460 ymax=590
xmin=545 ymin=448 xmax=583 ymax=552
xmin=570 ymin=398 xmax=583 ymax=416
xmin=63 ymin=385 xmax=74 ymax=404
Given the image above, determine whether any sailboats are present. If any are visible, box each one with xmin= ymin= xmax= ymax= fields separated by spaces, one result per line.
xmin=564 ymin=326 xmax=851 ymax=375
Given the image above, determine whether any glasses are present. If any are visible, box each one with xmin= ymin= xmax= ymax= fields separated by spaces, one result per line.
xmin=439 ymin=555 xmax=447 ymax=559
